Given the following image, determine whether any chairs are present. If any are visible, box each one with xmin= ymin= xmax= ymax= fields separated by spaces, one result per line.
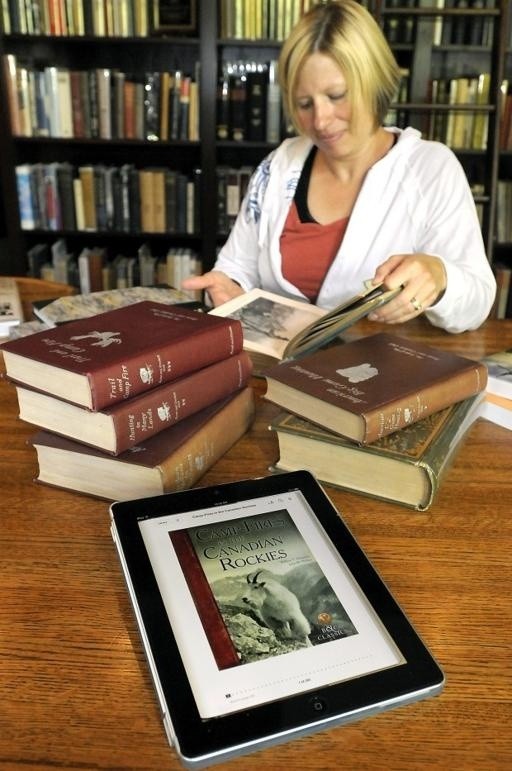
xmin=0 ymin=274 xmax=76 ymax=349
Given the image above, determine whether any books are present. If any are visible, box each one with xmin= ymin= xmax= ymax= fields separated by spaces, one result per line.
xmin=24 ymin=385 xmax=262 ymax=505
xmin=0 ymin=275 xmax=26 ymax=336
xmin=217 ymin=166 xmax=256 ymax=235
xmin=201 ymin=279 xmax=407 ymax=365
xmin=2 ymin=54 xmax=199 ymax=142
xmin=470 ymin=344 xmax=512 ymax=402
xmin=14 ymin=162 xmax=194 ymax=234
xmin=380 ymin=67 xmax=491 ymax=149
xmin=470 ymin=185 xmax=485 ymax=229
xmin=500 ymin=267 xmax=511 ymax=321
xmin=256 ymin=329 xmax=490 ymax=448
xmin=218 ymin=59 xmax=299 ymax=143
xmin=263 ymin=388 xmax=489 ymax=516
xmin=0 ymin=296 xmax=244 ymax=415
xmin=376 ymin=1 xmax=492 ymax=46
xmin=7 ymin=352 xmax=254 ymax=456
xmin=221 ymin=1 xmax=313 ymax=41
xmin=1 ymin=1 xmax=160 ymax=37
xmin=28 ymin=278 xmax=206 ymax=329
xmin=475 ymin=392 xmax=512 ymax=434
xmin=0 ymin=244 xmax=203 ymax=289
xmin=489 ymin=265 xmax=500 ymax=322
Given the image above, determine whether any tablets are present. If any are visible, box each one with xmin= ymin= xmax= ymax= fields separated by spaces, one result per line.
xmin=108 ymin=470 xmax=446 ymax=769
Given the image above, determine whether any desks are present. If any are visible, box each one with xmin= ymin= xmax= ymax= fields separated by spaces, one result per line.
xmin=0 ymin=305 xmax=511 ymax=771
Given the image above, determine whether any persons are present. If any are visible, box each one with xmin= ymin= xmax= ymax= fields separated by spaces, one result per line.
xmin=178 ymin=0 xmax=500 ymax=334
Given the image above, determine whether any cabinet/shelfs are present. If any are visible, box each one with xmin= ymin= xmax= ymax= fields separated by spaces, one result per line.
xmin=1 ymin=0 xmax=209 ymax=308
xmin=209 ymin=1 xmax=432 ymax=304
xmin=431 ymin=0 xmax=511 ymax=318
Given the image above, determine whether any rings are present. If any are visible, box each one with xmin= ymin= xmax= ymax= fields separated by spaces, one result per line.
xmin=410 ymin=296 xmax=421 ymax=311
